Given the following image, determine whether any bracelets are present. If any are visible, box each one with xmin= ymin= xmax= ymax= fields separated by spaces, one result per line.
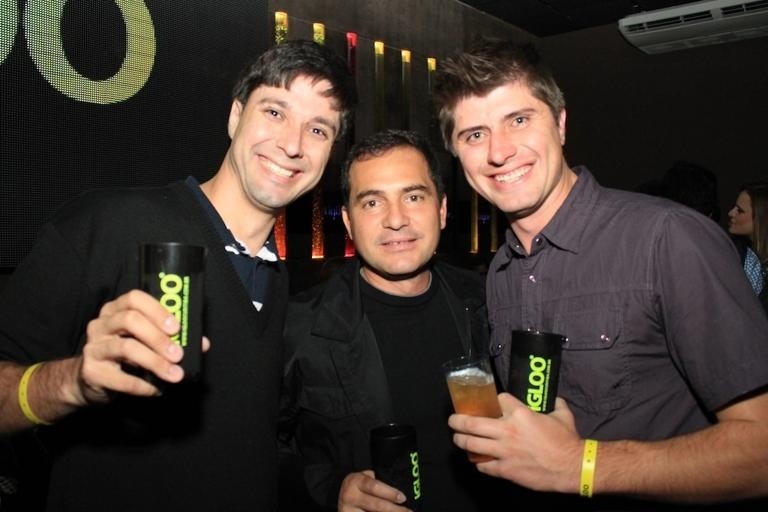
xmin=580 ymin=439 xmax=597 ymax=497
xmin=18 ymin=361 xmax=52 ymax=425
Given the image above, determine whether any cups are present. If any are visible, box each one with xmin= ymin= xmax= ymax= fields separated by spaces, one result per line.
xmin=508 ymin=329 xmax=570 ymax=417
xmin=368 ymin=421 xmax=425 ymax=512
xmin=139 ymin=240 xmax=204 ymax=385
xmin=444 ymin=353 xmax=505 ymax=466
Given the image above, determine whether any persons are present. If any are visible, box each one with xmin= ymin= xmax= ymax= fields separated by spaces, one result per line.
xmin=729 ymin=182 xmax=767 ymax=312
xmin=282 ymin=130 xmax=503 ymax=512
xmin=0 ymin=40 xmax=358 ymax=512
xmin=433 ymin=40 xmax=767 ymax=512
